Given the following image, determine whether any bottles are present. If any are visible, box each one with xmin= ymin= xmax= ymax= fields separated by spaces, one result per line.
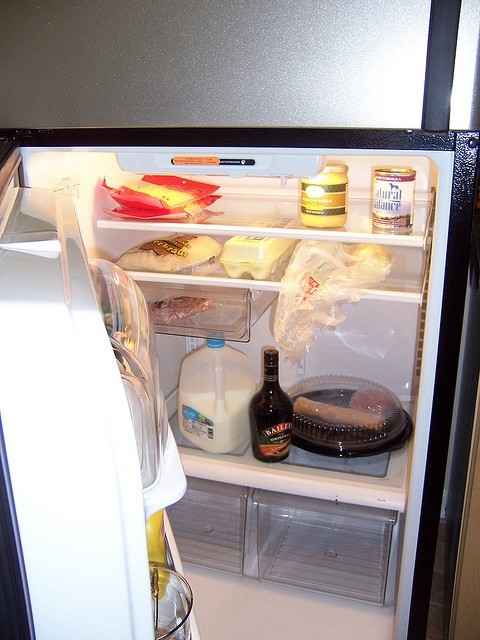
xmin=249 ymin=348 xmax=295 ymax=463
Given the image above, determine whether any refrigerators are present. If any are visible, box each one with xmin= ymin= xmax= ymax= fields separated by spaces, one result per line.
xmin=2 ymin=0 xmax=479 ymax=640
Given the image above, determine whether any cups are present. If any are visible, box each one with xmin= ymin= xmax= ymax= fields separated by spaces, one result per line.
xmin=148 ymin=559 xmax=193 ymax=640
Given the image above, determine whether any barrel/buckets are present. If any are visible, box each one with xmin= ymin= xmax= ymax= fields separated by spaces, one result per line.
xmin=177 ymin=333 xmax=257 ymax=453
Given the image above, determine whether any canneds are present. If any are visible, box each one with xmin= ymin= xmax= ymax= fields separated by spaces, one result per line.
xmin=298 ymin=165 xmax=348 ymax=228
xmin=370 ymin=165 xmax=415 ymax=233
